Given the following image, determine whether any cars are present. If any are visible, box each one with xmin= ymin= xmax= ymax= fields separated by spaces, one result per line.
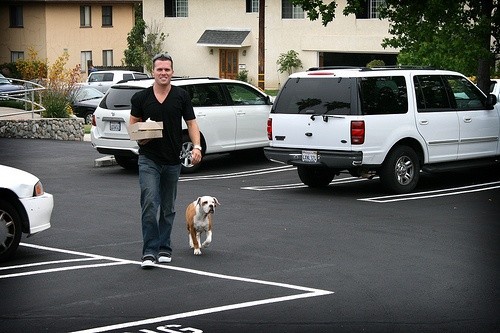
xmin=68 ymin=86 xmax=105 ymax=125
xmin=0 ymin=163 xmax=54 ymax=262
xmin=455 ymin=79 xmax=499 ymax=99
xmin=0 ymin=73 xmax=26 ymax=101
xmin=24 ymin=78 xmax=67 ymax=97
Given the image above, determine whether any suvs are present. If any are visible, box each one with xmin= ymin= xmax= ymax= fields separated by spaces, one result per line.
xmin=264 ymin=64 xmax=499 ymax=194
xmin=70 ymin=72 xmax=150 ymax=99
xmin=91 ymin=75 xmax=276 ymax=174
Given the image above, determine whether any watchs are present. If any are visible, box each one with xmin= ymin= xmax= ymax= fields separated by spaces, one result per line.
xmin=192 ymin=145 xmax=202 ymax=151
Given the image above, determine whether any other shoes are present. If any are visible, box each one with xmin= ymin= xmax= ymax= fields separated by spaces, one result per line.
xmin=157 ymin=252 xmax=171 ymax=263
xmin=140 ymin=256 xmax=155 ymax=268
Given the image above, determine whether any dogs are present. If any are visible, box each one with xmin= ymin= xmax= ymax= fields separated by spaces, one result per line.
xmin=184 ymin=194 xmax=221 ymax=256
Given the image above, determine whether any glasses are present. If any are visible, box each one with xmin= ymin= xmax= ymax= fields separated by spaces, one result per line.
xmin=151 ymin=53 xmax=173 ymax=70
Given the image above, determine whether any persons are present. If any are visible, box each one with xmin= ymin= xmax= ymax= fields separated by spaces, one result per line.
xmin=129 ymin=53 xmax=202 ymax=268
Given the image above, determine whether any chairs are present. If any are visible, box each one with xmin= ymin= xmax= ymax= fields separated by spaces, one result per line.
xmin=378 ymin=86 xmax=399 ymax=113
xmin=205 ymin=91 xmax=223 ymax=106
xmin=419 ymin=87 xmax=437 ymax=109
